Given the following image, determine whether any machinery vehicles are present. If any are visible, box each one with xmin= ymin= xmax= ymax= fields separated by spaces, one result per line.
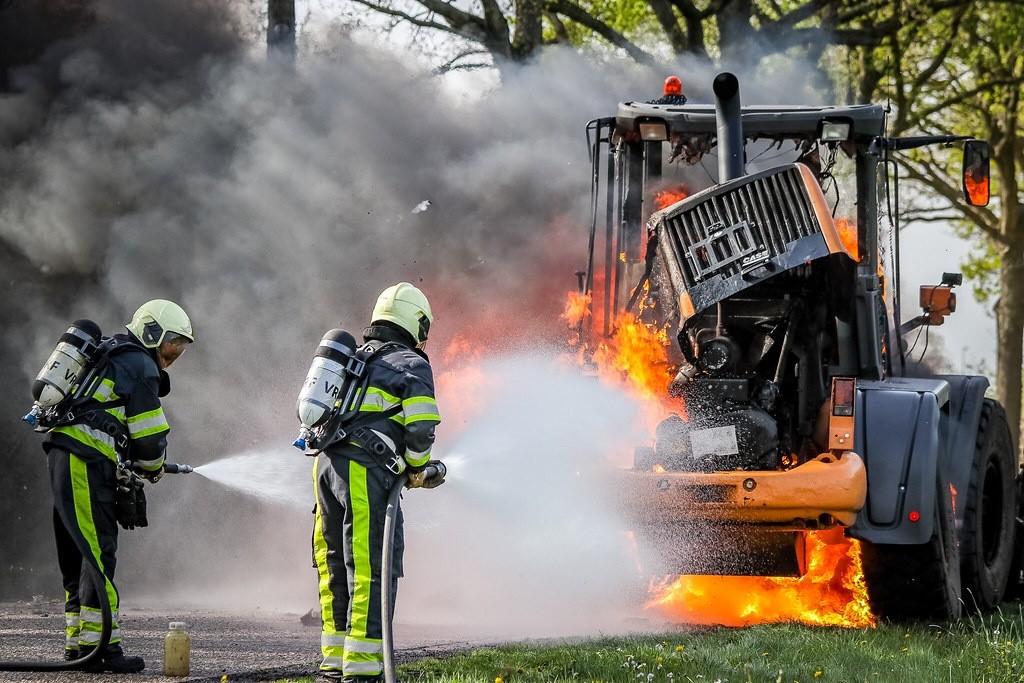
xmin=574 ymin=69 xmax=1024 ymax=630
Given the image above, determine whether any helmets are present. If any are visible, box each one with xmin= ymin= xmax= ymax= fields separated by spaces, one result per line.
xmin=125 ymin=298 xmax=194 ymax=370
xmin=368 ymin=281 xmax=433 ymax=349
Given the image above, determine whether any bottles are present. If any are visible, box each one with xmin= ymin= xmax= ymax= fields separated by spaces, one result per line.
xmin=164 ymin=622 xmax=190 ymax=677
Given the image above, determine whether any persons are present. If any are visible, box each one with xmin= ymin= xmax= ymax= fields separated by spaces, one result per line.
xmin=42 ymin=300 xmax=196 ymax=674
xmin=312 ymin=282 xmax=445 ymax=683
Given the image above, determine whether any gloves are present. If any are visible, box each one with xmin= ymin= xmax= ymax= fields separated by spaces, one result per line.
xmin=117 ymin=481 xmax=148 ymax=530
xmin=144 ymin=465 xmax=165 ymax=485
xmin=409 ymin=470 xmax=429 ymax=488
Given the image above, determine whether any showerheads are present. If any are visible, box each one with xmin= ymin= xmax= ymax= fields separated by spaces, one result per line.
xmin=420 ymin=460 xmax=447 ymax=489
xmin=164 ymin=463 xmax=193 ymax=474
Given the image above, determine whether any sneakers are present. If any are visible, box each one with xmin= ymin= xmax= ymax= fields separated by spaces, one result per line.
xmin=319 ymin=670 xmax=401 ymax=683
xmin=64 ymin=646 xmax=145 ymax=673
